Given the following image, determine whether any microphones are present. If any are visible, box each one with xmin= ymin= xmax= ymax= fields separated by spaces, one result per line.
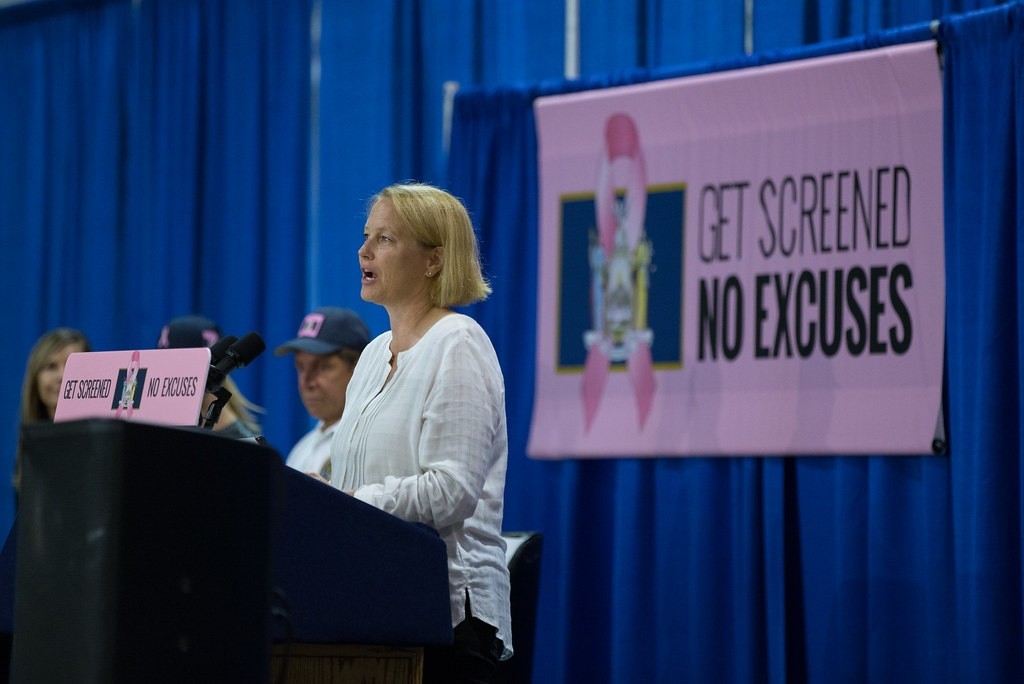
xmin=203 ymin=334 xmax=267 ymax=393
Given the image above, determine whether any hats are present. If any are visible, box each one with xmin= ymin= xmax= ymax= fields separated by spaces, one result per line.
xmin=274 ymin=308 xmax=370 ymax=356
xmin=156 ymin=316 xmax=222 ymax=349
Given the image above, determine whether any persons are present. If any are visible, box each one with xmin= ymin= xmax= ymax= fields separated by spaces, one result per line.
xmin=274 ymin=310 xmax=372 ymax=483
xmin=0 ymin=329 xmax=93 ymax=684
xmin=155 ymin=318 xmax=263 ymax=444
xmin=302 ymin=186 xmax=512 ymax=684
